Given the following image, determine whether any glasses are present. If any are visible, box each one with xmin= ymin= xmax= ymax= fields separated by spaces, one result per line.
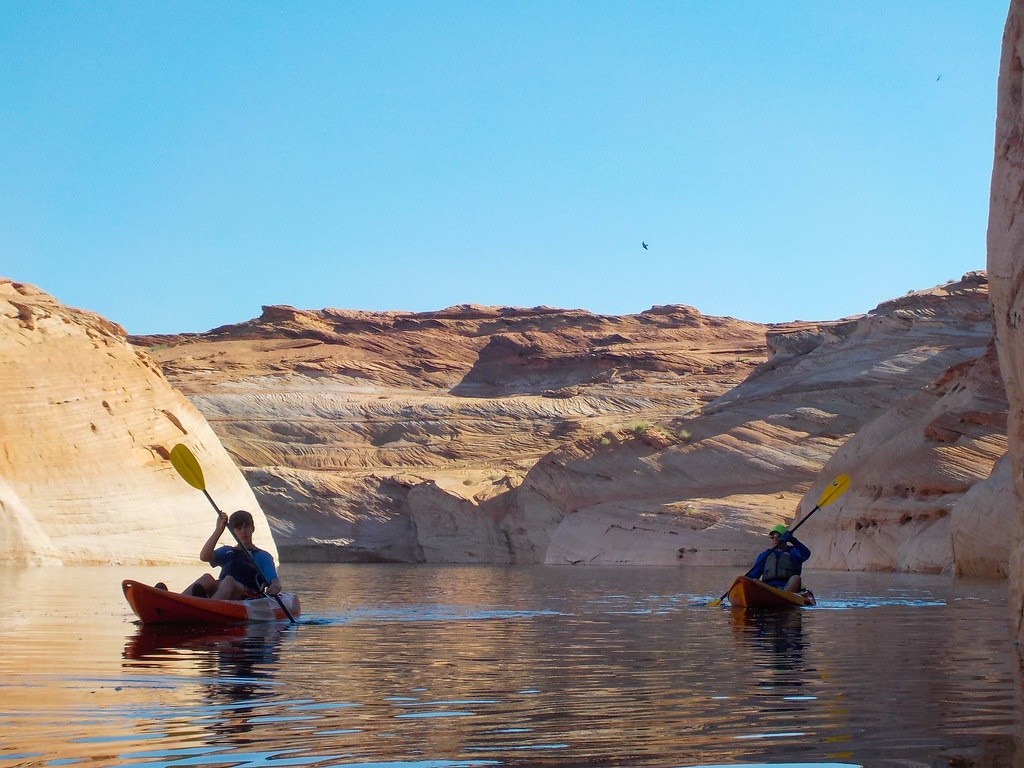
xmin=770 ymin=535 xmax=781 ymax=539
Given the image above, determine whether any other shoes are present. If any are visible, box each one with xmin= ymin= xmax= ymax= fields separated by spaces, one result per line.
xmin=154 ymin=582 xmax=168 ymax=591
xmin=192 ymin=583 xmax=207 ymax=598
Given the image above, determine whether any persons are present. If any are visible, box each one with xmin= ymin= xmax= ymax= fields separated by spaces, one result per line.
xmin=746 ymin=524 xmax=811 ymax=593
xmin=154 ymin=510 xmax=282 ymax=600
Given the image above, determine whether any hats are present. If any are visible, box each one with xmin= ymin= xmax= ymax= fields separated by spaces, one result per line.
xmin=769 ymin=524 xmax=787 ymax=535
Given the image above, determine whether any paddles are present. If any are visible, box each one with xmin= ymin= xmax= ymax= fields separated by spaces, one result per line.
xmin=168 ymin=443 xmax=296 ymax=624
xmin=709 ymin=472 xmax=853 ymax=608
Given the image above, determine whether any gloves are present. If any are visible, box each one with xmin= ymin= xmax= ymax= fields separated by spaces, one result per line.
xmin=781 ymin=531 xmax=793 ymax=541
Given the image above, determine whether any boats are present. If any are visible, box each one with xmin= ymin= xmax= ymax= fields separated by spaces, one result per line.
xmin=727 ymin=575 xmax=808 ymax=608
xmin=122 ymin=579 xmax=301 ymax=626
